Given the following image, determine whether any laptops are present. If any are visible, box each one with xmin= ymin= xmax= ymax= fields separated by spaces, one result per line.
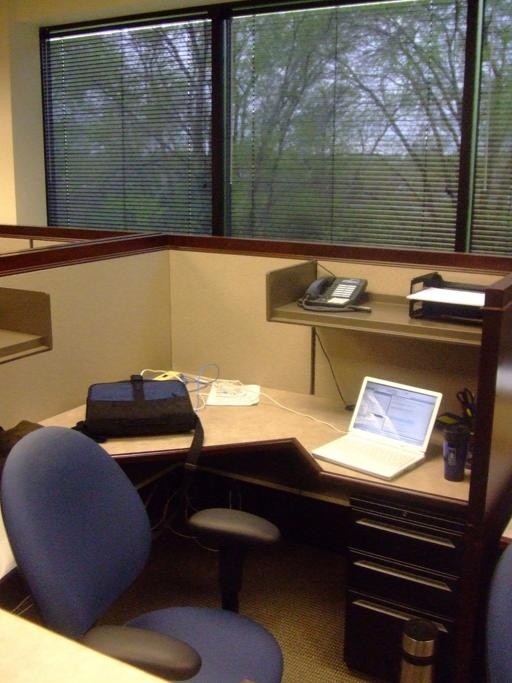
xmin=313 ymin=376 xmax=443 ymax=480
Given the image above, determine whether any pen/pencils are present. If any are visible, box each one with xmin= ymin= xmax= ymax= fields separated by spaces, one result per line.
xmin=465 ymin=408 xmax=477 ymax=416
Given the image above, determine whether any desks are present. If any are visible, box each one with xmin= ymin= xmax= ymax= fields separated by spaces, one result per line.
xmin=0 ymin=233 xmax=511 ymax=683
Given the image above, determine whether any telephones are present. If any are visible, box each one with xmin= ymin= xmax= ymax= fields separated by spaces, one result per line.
xmin=297 ymin=274 xmax=368 ymax=308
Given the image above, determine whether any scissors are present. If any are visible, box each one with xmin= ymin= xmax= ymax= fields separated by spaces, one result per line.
xmin=456 ymin=388 xmax=474 ymax=406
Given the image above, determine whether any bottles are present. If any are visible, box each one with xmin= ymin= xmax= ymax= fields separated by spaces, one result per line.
xmin=397 ymin=619 xmax=438 ymax=683
xmin=442 ymin=424 xmax=467 ymax=482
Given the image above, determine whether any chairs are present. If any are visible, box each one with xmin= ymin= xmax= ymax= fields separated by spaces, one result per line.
xmin=487 ymin=545 xmax=512 ymax=682
xmin=3 ymin=426 xmax=283 ymax=682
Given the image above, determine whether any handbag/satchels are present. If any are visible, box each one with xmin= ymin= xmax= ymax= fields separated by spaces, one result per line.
xmin=85 ymin=374 xmax=195 ymax=437
